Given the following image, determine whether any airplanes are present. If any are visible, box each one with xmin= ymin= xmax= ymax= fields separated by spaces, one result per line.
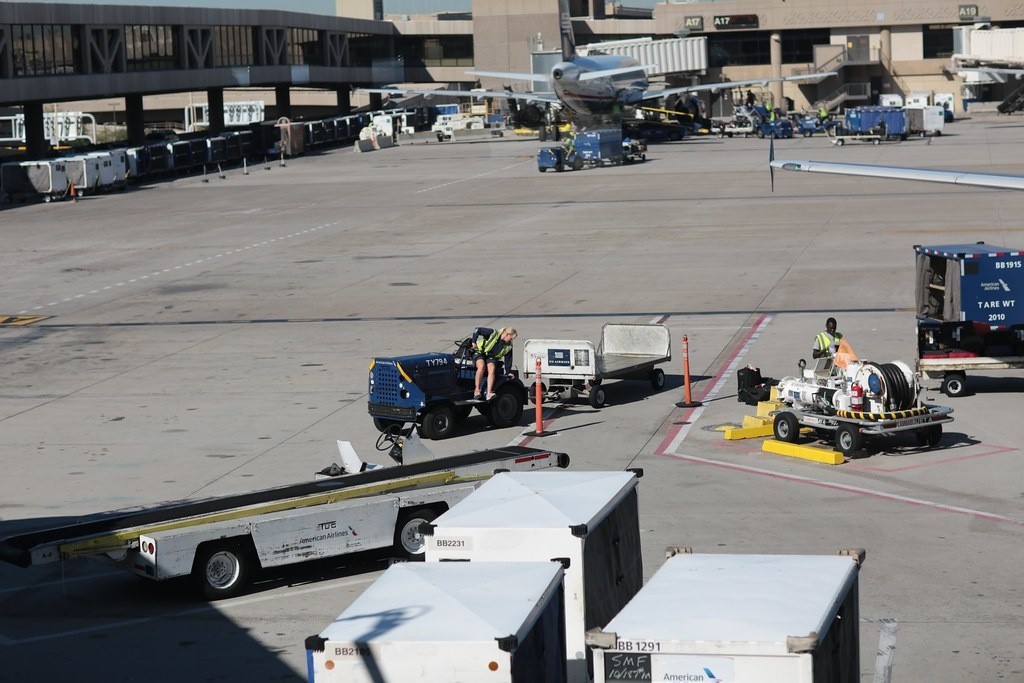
xmin=358 ymin=54 xmax=840 ymax=143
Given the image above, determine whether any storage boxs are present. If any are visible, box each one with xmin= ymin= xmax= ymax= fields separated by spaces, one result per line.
xmin=844 ymin=106 xmax=906 ymax=135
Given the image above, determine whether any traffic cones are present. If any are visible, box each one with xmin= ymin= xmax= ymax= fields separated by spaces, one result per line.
xmin=70 ymin=182 xmax=77 ymax=197
xmin=106 ymin=102 xmax=120 ymax=132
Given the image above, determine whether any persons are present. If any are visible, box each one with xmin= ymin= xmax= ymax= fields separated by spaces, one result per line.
xmin=745 ymin=90 xmax=755 ymax=106
xmin=812 ymin=317 xmax=844 ymax=359
xmin=772 ymin=107 xmax=785 ymax=120
xmin=470 ymin=326 xmax=517 ymax=401
xmin=817 ymin=103 xmax=832 ymax=125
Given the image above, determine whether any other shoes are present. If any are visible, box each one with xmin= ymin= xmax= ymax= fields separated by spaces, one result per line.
xmin=485 ymin=391 xmax=497 ymax=401
xmin=474 ymin=389 xmax=482 ymax=398
